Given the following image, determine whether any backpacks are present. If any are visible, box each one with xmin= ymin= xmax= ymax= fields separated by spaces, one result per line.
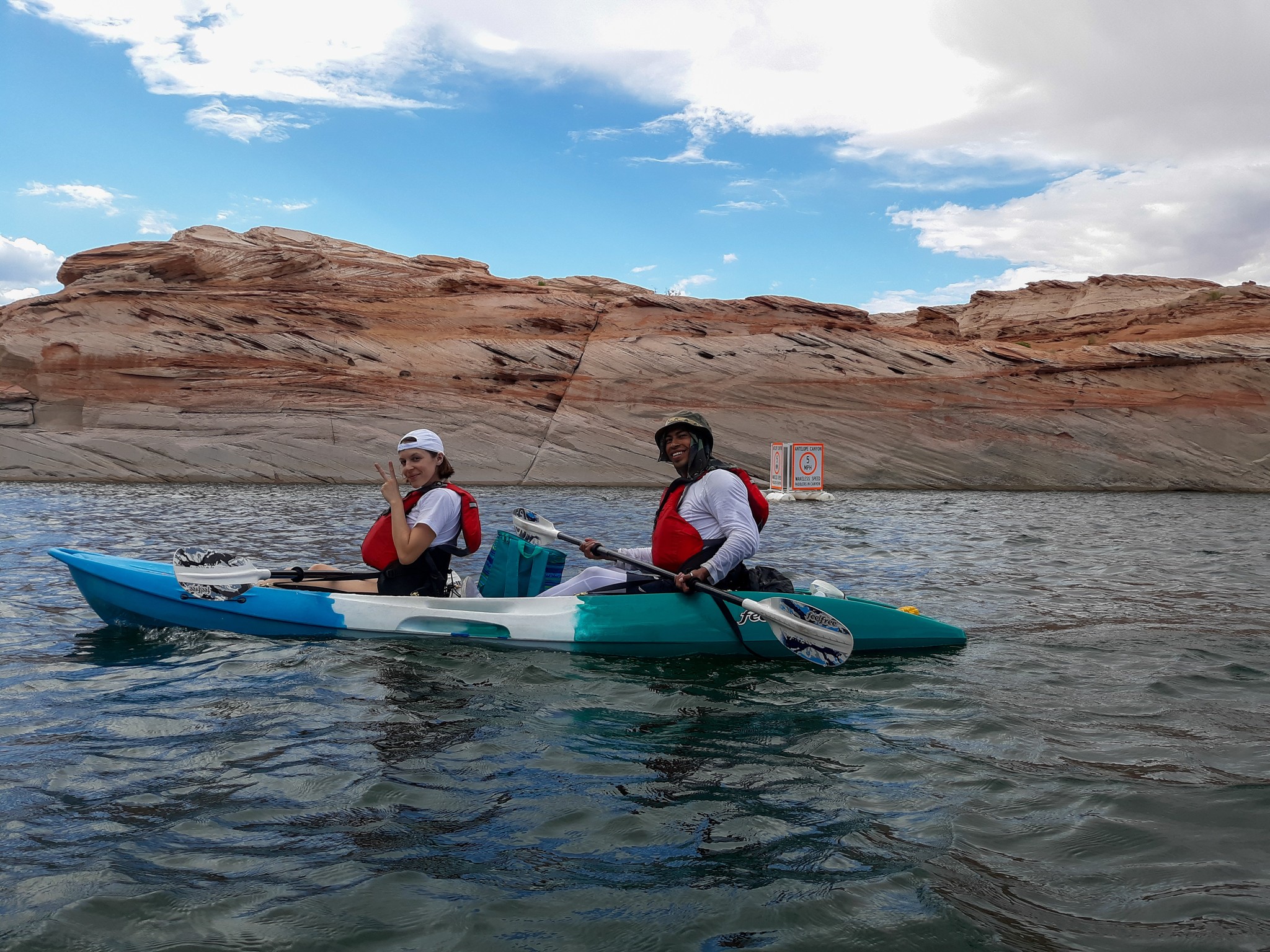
xmin=475 ymin=528 xmax=568 ymax=597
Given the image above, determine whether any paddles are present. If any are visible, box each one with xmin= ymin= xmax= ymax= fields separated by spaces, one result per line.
xmin=171 ymin=547 xmax=461 ymax=606
xmin=507 ymin=500 xmax=856 ymax=673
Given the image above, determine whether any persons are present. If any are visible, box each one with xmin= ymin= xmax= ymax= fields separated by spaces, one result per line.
xmin=461 ymin=410 xmax=760 ymax=598
xmin=254 ymin=429 xmax=482 ymax=597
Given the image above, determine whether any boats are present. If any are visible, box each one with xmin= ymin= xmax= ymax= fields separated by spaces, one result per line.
xmin=41 ymin=544 xmax=968 ymax=663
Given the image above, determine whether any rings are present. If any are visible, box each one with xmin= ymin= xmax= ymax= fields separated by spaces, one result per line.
xmin=688 ymin=572 xmax=693 ymax=578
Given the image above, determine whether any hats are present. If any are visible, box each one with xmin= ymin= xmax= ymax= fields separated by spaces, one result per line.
xmin=397 ymin=429 xmax=456 ymax=480
xmin=654 ymin=410 xmax=738 ymax=481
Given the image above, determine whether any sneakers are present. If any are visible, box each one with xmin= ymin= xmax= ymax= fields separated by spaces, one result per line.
xmin=461 ymin=576 xmax=483 ymax=598
